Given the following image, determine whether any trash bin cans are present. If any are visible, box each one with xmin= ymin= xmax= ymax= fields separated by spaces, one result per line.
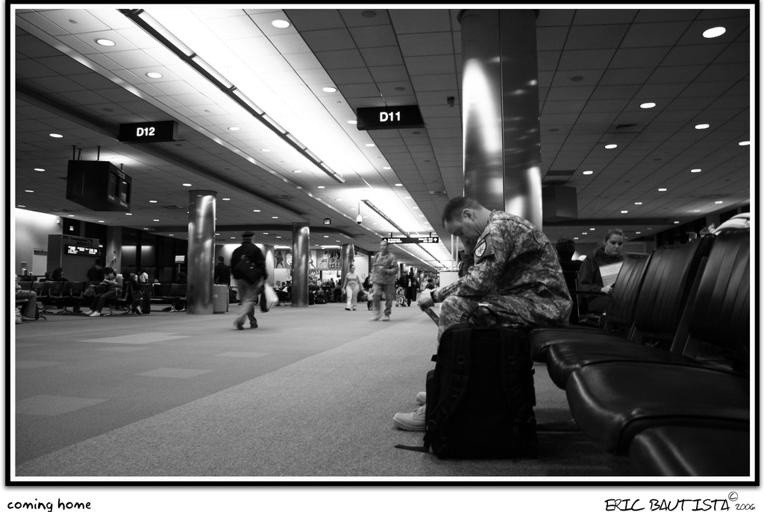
xmin=212 ymin=284 xmax=229 ymax=312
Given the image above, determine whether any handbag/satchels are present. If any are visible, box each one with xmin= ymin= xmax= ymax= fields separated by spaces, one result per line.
xmin=425 ymin=306 xmax=543 ymax=462
xmin=235 ymin=252 xmax=261 ymax=284
xmin=259 ymin=283 xmax=278 ymax=315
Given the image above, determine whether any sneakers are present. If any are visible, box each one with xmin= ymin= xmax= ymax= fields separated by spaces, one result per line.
xmin=391 ymin=391 xmax=430 ymax=431
xmin=234 ymin=321 xmax=258 ymax=330
xmin=84 ymin=310 xmax=101 ymax=317
xmin=370 ymin=314 xmax=390 ymax=321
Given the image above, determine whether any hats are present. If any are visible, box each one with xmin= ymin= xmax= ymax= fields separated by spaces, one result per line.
xmin=241 ymin=231 xmax=256 ymax=239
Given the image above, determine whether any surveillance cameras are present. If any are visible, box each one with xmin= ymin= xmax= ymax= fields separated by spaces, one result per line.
xmin=357 ymin=215 xmax=362 ymax=225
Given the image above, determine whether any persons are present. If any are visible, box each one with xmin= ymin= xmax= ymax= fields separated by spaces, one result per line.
xmin=15 ymin=261 xmax=149 ymax=320
xmin=391 ymin=197 xmax=574 ymax=434
xmin=230 ymin=231 xmax=266 ymax=330
xmin=572 ymin=226 xmax=626 ymax=315
xmin=279 ymin=237 xmax=438 ymax=320
xmin=555 ymin=239 xmax=582 ymax=271
xmin=214 ymin=255 xmax=230 ymax=286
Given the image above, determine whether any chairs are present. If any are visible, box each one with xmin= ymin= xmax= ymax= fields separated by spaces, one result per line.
xmin=532 ymin=232 xmax=749 ymax=477
xmin=15 ymin=280 xmax=185 ymax=321
